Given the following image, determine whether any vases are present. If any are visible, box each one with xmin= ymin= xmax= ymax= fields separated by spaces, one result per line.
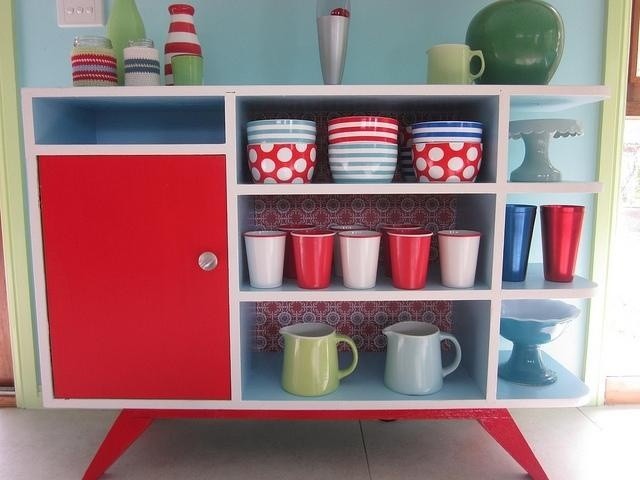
xmin=498 ymin=303 xmax=579 ymax=387
xmin=466 ymin=2 xmax=563 ymax=85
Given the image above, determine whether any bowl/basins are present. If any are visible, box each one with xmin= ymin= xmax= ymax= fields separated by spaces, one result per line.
xmin=241 ymin=114 xmax=484 ymax=184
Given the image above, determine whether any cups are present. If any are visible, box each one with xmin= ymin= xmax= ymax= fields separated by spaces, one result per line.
xmin=72 ymin=34 xmax=118 ymax=87
xmin=170 ymin=53 xmax=206 ymax=86
xmin=275 ymin=221 xmax=434 ymax=289
xmin=243 ymin=229 xmax=289 ymax=290
xmin=539 ymin=204 xmax=587 ymax=283
xmin=123 ymin=37 xmax=162 ymax=86
xmin=435 ymin=228 xmax=482 ymax=289
xmin=501 ymin=203 xmax=538 ymax=283
xmin=316 ymin=0 xmax=350 ymax=85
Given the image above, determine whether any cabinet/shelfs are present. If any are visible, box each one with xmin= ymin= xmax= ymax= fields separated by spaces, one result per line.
xmin=14 ymin=84 xmax=616 ymax=480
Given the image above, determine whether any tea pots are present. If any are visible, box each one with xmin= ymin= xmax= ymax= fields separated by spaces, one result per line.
xmin=277 ymin=322 xmax=359 ymax=397
xmin=424 ymin=44 xmax=488 ymax=84
xmin=380 ymin=319 xmax=463 ymax=397
